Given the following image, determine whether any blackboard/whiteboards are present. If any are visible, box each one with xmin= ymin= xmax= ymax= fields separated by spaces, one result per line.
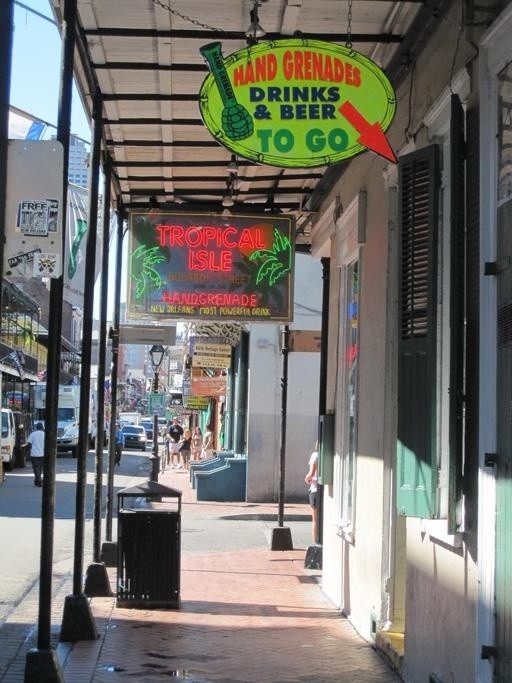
xmin=125 ymin=209 xmax=296 ymax=322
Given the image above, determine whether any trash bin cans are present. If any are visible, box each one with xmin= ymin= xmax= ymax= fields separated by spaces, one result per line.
xmin=116 ymin=481 xmax=182 ymax=609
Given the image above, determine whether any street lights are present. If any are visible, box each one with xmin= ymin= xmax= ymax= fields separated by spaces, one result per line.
xmin=145 ymin=344 xmax=168 ymax=500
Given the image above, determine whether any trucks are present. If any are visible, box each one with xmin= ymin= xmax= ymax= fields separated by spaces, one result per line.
xmin=31 ymin=383 xmax=96 ymax=457
xmin=1 ymin=407 xmax=32 ymax=473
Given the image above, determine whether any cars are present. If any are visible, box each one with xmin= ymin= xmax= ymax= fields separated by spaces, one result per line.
xmin=110 ymin=410 xmax=170 ymax=450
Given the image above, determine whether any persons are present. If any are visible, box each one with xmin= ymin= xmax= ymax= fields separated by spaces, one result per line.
xmin=26 ymin=421 xmax=45 ymax=486
xmin=162 ymin=416 xmax=215 ymax=471
xmin=114 ymin=421 xmax=125 ymax=467
xmin=305 ymin=438 xmax=319 ymax=545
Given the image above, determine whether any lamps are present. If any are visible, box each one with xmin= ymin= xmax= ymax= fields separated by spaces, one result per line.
xmin=222 ymin=154 xmax=240 ymax=207
xmin=246 ymin=10 xmax=267 ymax=39
